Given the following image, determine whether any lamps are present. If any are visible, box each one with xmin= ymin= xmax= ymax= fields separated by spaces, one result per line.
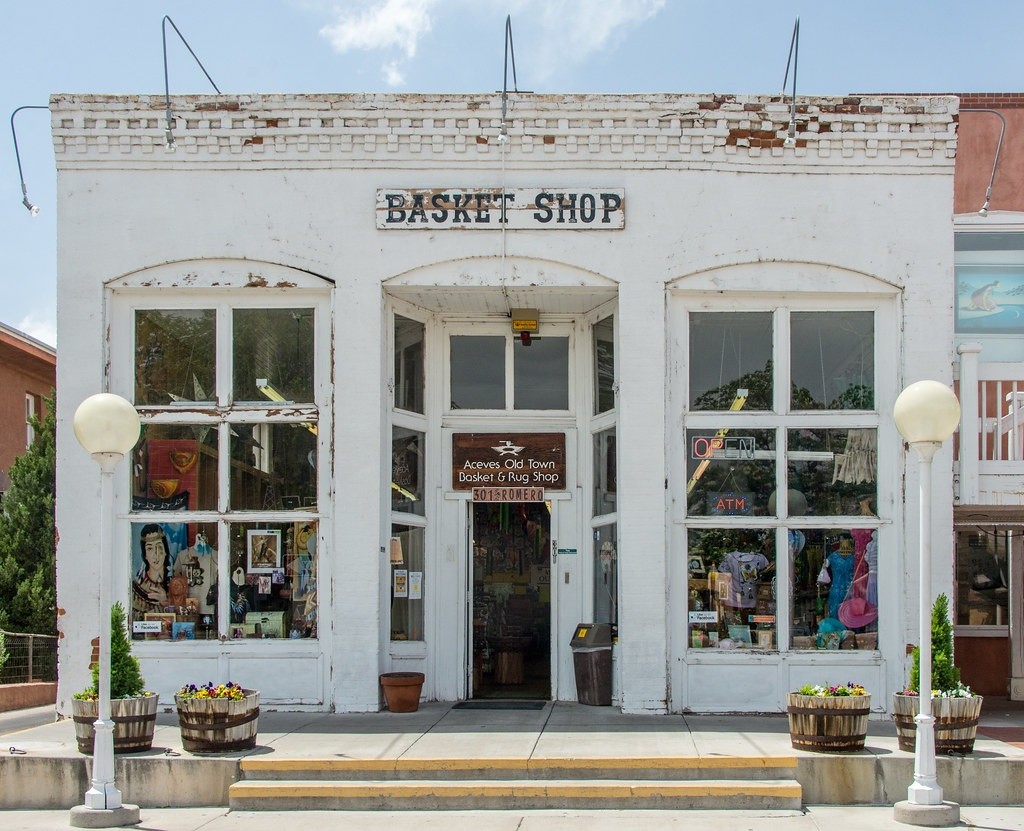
xmin=161 ymin=15 xmax=221 ymax=154
xmin=12 ymin=106 xmax=50 ymax=218
xmin=687 ymin=318 xmax=748 ymax=494
xmin=256 ymin=378 xmax=317 ymax=436
xmin=780 ymin=15 xmax=800 ymax=149
xmin=959 ymin=109 xmax=1006 ymax=217
xmin=495 ymin=14 xmax=534 ymax=143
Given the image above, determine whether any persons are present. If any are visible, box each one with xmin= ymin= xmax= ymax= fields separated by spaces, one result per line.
xmin=849 ymin=498 xmax=877 ymax=601
xmin=717 ymin=542 xmax=770 ymax=642
xmin=863 ymin=529 xmax=878 ymax=633
xmin=824 ymin=538 xmax=856 ymax=621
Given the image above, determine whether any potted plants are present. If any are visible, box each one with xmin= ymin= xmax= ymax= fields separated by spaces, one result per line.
xmin=893 ymin=592 xmax=984 ymax=755
xmin=71 ymin=601 xmax=159 ymax=755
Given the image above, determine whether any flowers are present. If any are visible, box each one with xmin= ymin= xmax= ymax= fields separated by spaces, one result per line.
xmin=903 ymin=681 xmax=981 ymax=699
xmin=77 ymin=690 xmax=150 ymax=699
xmin=177 ymin=681 xmax=245 ymax=701
xmin=796 ymin=680 xmax=864 ymax=696
xmin=815 ymin=632 xmax=847 ymax=649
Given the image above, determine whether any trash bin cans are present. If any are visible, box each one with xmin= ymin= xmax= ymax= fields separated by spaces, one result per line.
xmin=569 ymin=623 xmax=613 ymax=707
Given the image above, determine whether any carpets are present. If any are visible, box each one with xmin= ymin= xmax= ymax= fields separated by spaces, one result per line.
xmin=452 ymin=702 xmax=547 ymax=709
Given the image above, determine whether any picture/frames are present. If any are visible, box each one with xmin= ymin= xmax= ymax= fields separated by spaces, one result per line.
xmin=247 ymin=529 xmax=282 ymax=574
xmin=144 ymin=612 xmax=177 ymax=640
xmin=728 ymin=625 xmax=752 ymax=647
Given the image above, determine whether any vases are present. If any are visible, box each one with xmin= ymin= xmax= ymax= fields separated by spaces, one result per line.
xmin=174 ymin=689 xmax=259 ymax=753
xmin=380 ymin=672 xmax=425 ymax=712
xmin=787 ymin=693 xmax=871 ymax=752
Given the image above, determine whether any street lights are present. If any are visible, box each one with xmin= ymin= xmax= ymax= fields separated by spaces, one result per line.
xmin=892 ymin=380 xmax=961 ymax=805
xmin=73 ymin=394 xmax=140 ymax=811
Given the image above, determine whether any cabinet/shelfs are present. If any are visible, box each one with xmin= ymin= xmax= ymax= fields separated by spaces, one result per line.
xmin=146 ymin=439 xmax=270 ymax=545
